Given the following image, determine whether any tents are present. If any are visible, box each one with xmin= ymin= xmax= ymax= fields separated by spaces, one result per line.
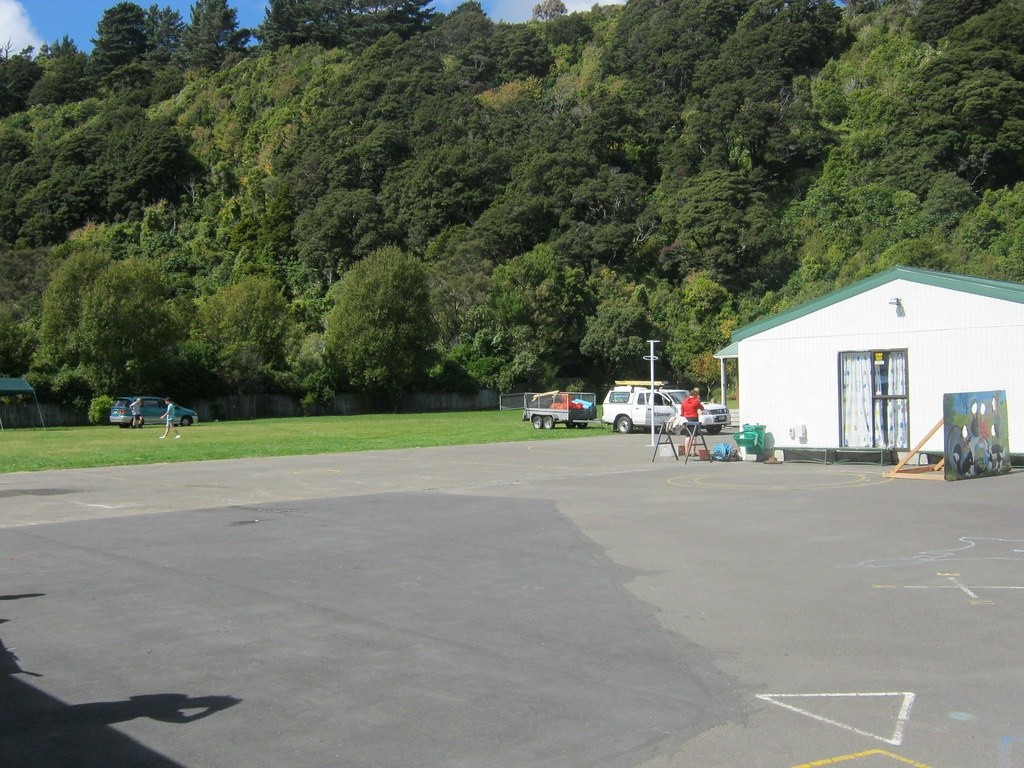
xmin=0 ymin=378 xmax=45 ymax=431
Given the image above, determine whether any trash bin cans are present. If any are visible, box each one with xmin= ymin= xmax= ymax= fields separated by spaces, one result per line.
xmin=733 ymin=423 xmax=766 ymax=454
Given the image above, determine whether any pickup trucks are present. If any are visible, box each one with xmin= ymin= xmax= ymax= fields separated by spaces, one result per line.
xmin=603 ymin=380 xmax=731 ymax=435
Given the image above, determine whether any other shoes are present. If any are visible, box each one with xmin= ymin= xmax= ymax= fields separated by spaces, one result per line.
xmin=160 ymin=436 xmax=166 ymax=439
xmin=175 ymin=435 xmax=181 ymax=438
xmin=685 ymin=452 xmax=698 ymax=457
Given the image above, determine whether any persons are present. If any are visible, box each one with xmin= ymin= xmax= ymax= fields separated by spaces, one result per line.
xmin=159 ymin=397 xmax=180 ymax=438
xmin=681 ymin=391 xmax=704 ymax=457
xmin=694 ymin=387 xmax=701 ymax=401
xmin=130 ymin=398 xmax=142 ymax=428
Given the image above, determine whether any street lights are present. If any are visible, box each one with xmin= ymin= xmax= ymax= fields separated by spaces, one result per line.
xmin=643 ymin=339 xmax=661 ymax=448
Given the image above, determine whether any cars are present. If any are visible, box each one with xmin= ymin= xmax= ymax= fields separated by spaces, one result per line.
xmin=110 ymin=396 xmax=199 ymax=428
